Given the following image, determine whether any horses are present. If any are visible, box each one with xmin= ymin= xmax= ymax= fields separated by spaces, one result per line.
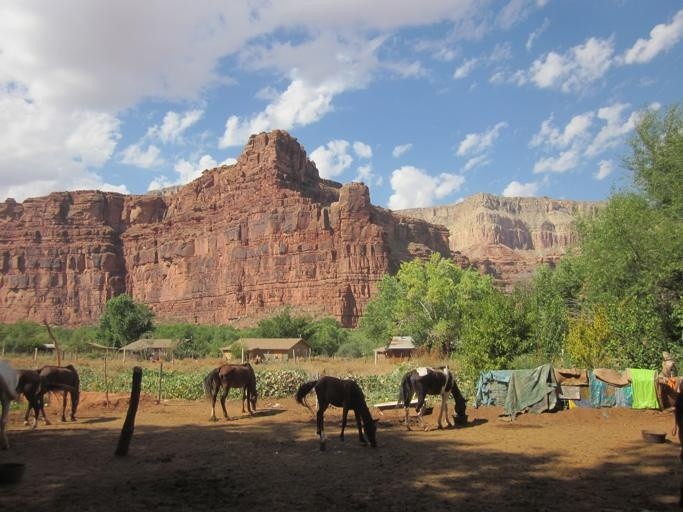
xmin=294 ymin=375 xmax=380 ymax=452
xmin=0 ymin=361 xmax=80 ymax=448
xmin=202 ymin=362 xmax=260 ymax=421
xmin=394 ymin=365 xmax=471 ymax=432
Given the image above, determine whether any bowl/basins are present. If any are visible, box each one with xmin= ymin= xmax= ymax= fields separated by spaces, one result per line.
xmin=417 ymin=407 xmax=434 ymax=415
xmin=0 ymin=463 xmax=26 ymax=482
xmin=640 ymin=430 xmax=667 ymax=445
xmin=453 ymin=414 xmax=468 ymax=427
